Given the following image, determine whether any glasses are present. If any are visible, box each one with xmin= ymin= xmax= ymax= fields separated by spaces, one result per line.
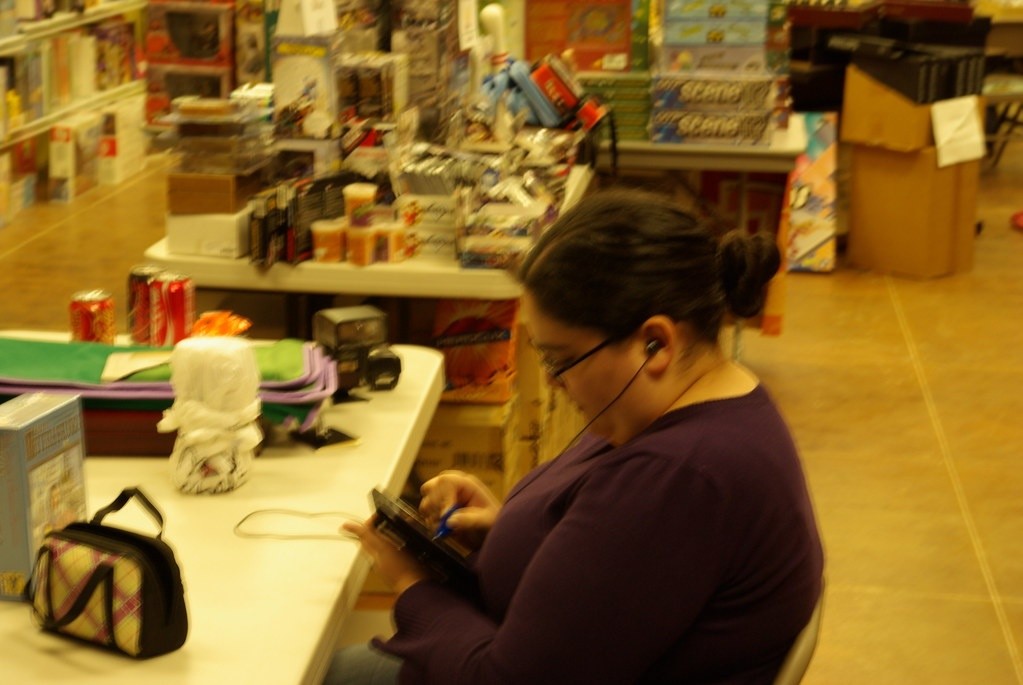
xmin=529 ymin=323 xmax=638 ymax=382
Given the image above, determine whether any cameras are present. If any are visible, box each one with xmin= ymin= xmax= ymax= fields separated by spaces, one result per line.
xmin=313 ymin=305 xmax=402 ymax=394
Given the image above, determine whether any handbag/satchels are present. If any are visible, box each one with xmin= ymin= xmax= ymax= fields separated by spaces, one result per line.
xmin=23 ymin=488 xmax=187 ymax=660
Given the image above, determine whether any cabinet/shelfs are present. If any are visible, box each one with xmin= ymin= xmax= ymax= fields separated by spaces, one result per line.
xmin=0 ymin=0 xmax=148 ymax=150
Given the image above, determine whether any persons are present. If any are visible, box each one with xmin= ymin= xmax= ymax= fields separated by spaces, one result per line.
xmin=325 ymin=195 xmax=823 ymax=685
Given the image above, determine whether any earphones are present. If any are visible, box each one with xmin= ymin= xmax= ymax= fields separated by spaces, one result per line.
xmin=646 ymin=338 xmax=663 ymax=358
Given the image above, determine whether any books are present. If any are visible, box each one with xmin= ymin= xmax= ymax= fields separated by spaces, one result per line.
xmin=0 ymin=0 xmax=147 ymax=220
xmin=248 ymin=170 xmax=364 ymax=265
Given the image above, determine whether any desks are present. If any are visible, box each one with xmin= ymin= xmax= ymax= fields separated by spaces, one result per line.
xmin=595 ymin=112 xmax=809 ymax=228
xmin=145 ymin=164 xmax=596 ymax=301
xmin=0 ymin=330 xmax=443 ymax=685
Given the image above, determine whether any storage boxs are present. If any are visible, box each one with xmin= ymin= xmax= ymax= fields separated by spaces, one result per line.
xmin=164 ymin=164 xmax=266 ymax=216
xmin=521 ymin=0 xmax=793 ymax=149
xmin=404 ymin=392 xmax=522 ymax=504
xmin=0 ymin=388 xmax=88 ymax=603
xmin=166 ymin=199 xmax=263 ymax=260
xmin=840 ymin=62 xmax=987 ymax=151
xmin=0 ymin=0 xmax=479 ymax=259
xmin=846 ymin=143 xmax=977 ymax=283
xmin=428 ymin=301 xmax=520 ymax=406
xmin=408 ymin=304 xmax=555 ymax=441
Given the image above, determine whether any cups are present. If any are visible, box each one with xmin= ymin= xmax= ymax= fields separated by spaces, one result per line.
xmin=309 ymin=216 xmax=407 ymax=265
xmin=343 ymin=183 xmax=380 ymax=227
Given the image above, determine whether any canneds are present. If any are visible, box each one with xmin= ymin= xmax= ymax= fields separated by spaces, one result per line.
xmin=125 ymin=262 xmax=195 ymax=347
xmin=69 ymin=288 xmax=115 ymax=345
